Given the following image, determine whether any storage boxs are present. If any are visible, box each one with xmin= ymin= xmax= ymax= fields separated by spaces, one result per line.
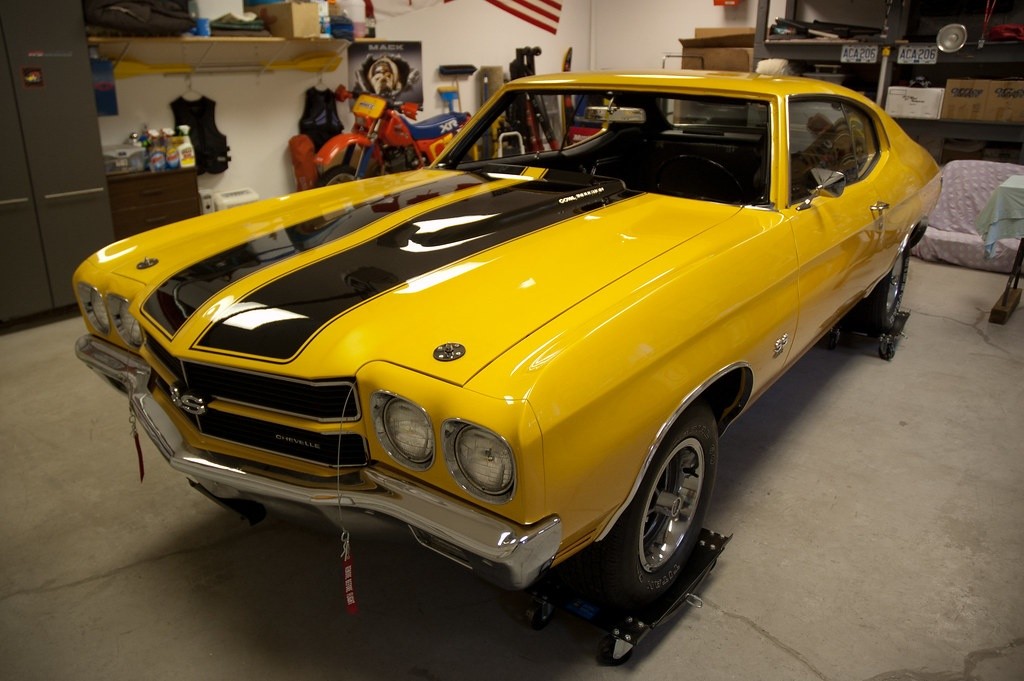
xmin=244 ymin=1 xmax=321 ymax=40
xmin=939 ymin=79 xmax=1024 ymax=124
xmin=884 ymin=85 xmax=945 ymax=122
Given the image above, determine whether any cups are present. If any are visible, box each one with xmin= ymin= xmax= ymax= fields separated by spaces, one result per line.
xmin=190 ymin=16 xmax=209 ymax=37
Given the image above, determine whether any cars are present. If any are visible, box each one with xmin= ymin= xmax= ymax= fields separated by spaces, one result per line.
xmin=69 ymin=65 xmax=948 ymax=662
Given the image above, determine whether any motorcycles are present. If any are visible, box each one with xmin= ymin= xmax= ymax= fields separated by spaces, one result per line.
xmin=309 ymin=69 xmax=485 ymax=191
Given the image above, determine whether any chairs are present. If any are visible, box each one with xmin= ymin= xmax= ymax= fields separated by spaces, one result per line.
xmin=580 ymin=127 xmax=766 ymax=206
